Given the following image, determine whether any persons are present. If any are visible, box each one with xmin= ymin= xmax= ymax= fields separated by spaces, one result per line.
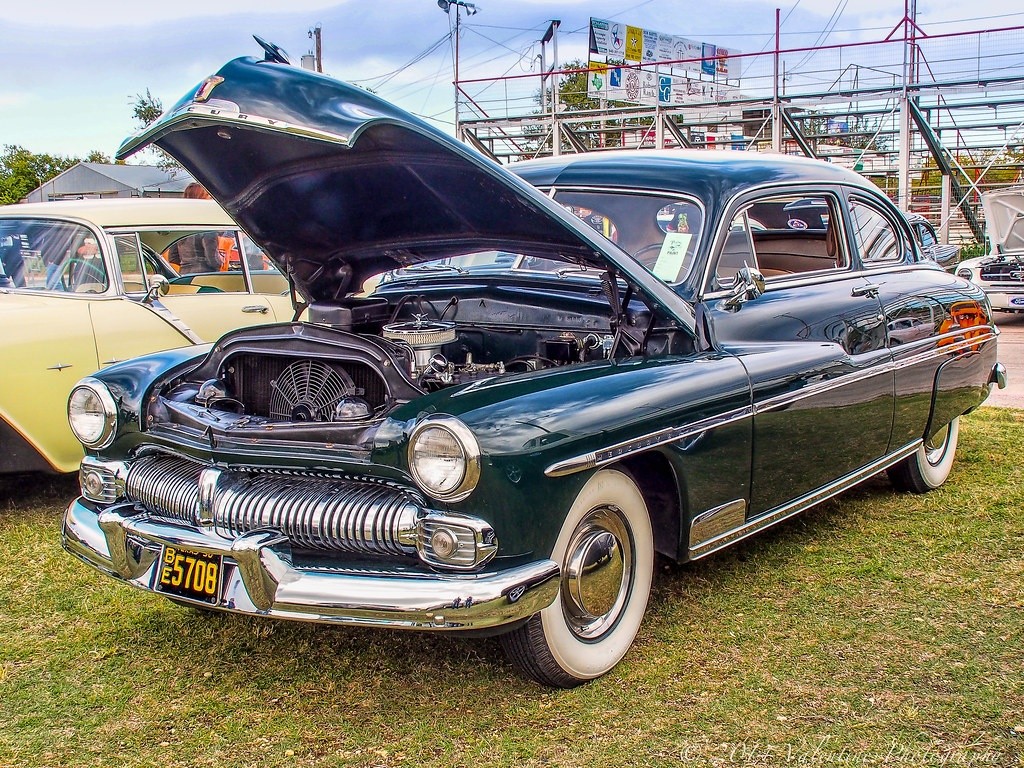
xmin=0 ymin=233 xmax=104 ymax=291
xmin=168 ymin=183 xmax=225 ymax=275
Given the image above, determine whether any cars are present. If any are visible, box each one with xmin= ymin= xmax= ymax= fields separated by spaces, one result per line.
xmin=956 ymin=182 xmax=1024 ymax=314
xmin=57 ymin=33 xmax=1008 ymax=691
xmin=0 ymin=198 xmax=385 ymax=491
